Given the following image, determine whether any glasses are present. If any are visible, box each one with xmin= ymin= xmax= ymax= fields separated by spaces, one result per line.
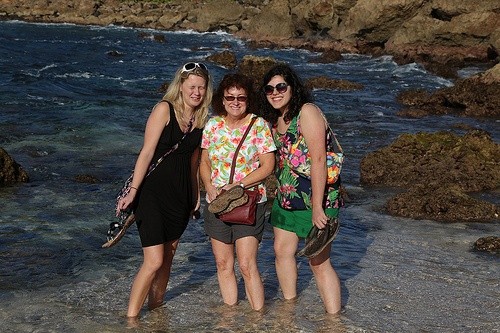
xmin=263 ymin=83 xmax=290 ymax=94
xmin=181 ymin=62 xmax=208 ymax=74
xmin=223 ymin=96 xmax=247 ymax=101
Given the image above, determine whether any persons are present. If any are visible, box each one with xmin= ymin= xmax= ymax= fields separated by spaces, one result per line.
xmin=202 ymin=72 xmax=277 ymax=311
xmin=255 ymin=65 xmax=343 ymax=314
xmin=115 ymin=62 xmax=214 ymax=318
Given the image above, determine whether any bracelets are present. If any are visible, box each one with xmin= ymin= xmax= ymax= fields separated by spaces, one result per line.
xmin=130 ymin=186 xmax=138 ymax=191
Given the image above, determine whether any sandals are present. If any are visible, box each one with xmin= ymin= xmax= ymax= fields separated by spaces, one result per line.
xmin=102 ymin=208 xmax=135 ymax=248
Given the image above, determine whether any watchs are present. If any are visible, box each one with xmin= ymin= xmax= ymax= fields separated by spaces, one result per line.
xmin=239 ymin=181 xmax=243 ymax=188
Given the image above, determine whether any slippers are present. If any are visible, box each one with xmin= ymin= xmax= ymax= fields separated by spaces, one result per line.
xmin=305 ymin=218 xmax=339 ymax=259
xmin=297 ymin=220 xmax=329 ymax=256
xmin=218 ymin=193 xmax=248 ymax=215
xmin=207 ymin=186 xmax=244 ymax=213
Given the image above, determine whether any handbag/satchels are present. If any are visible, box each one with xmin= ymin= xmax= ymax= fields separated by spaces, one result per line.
xmin=287 ymin=103 xmax=345 ymax=184
xmin=114 ymin=174 xmax=135 ymax=219
xmin=219 ymin=190 xmax=259 ymax=225
